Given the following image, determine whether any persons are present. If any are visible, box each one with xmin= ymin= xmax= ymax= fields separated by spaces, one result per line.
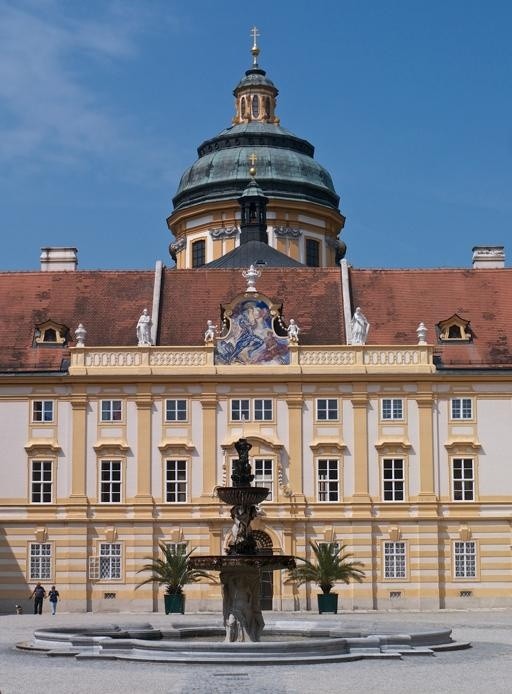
xmin=216 ymin=564 xmax=270 ymax=642
xmin=348 ymin=305 xmax=368 ymax=344
xmin=28 ymin=581 xmax=47 ymax=615
xmin=284 ymin=318 xmax=301 ymax=342
xmin=203 ymin=319 xmax=217 ymax=343
xmin=45 ymin=585 xmax=60 ymax=615
xmin=135 ymin=308 xmax=154 ymax=347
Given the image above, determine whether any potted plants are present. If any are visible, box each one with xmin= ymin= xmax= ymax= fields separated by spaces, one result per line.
xmin=132 ymin=539 xmax=217 ymax=615
xmin=282 ymin=537 xmax=365 ymax=615
